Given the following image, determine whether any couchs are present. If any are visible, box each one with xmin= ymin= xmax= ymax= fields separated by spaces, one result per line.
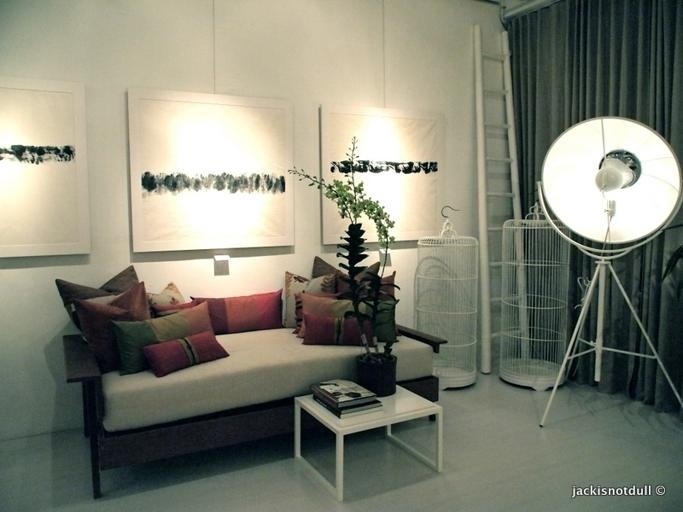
xmin=61 ymin=323 xmax=449 ymax=501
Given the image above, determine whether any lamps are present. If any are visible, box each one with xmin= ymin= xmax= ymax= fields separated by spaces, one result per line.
xmin=535 ymin=114 xmax=680 ymax=430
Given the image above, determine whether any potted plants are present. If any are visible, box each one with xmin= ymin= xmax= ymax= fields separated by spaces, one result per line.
xmin=287 ymin=135 xmax=402 ymax=398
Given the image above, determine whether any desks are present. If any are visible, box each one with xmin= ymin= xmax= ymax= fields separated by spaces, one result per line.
xmin=292 ymin=382 xmax=445 ymax=502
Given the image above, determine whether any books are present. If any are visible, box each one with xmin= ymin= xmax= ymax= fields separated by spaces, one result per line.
xmin=309 ymin=377 xmax=383 ymax=420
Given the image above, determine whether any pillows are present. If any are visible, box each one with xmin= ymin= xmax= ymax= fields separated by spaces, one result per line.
xmin=101 ymin=301 xmax=216 ymax=377
xmin=53 ymin=263 xmax=141 ymax=344
xmin=281 ymin=254 xmax=400 ymax=346
xmin=68 ymin=280 xmax=155 ymax=374
xmin=189 ymin=288 xmax=287 ymax=336
xmin=138 ymin=330 xmax=229 ymax=380
xmin=145 ymin=280 xmax=187 ymax=307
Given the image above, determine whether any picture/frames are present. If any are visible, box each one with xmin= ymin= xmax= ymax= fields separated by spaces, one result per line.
xmin=318 ymin=103 xmax=447 ymax=247
xmin=0 ymin=75 xmax=92 ymax=260
xmin=125 ymin=86 xmax=295 ymax=253
